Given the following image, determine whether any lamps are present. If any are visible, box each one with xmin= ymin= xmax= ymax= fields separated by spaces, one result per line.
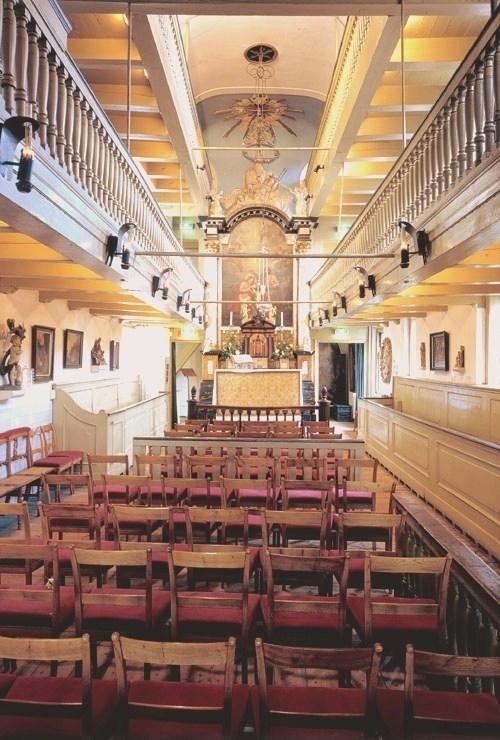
xmin=215 ymin=44 xmax=304 ymax=178
xmin=191 ymin=306 xmax=201 ymax=319
xmin=354 ymin=266 xmax=376 ymax=298
xmin=177 ymin=289 xmax=191 ymax=313
xmin=0 ymin=116 xmax=40 ymax=192
xmin=105 ymin=223 xmax=136 ymax=270
xmin=332 ymin=291 xmax=347 ymax=316
xmin=318 ymin=308 xmax=330 ymax=326
xmin=398 ymin=221 xmax=431 ymax=269
xmin=151 ymin=267 xmax=173 ymax=300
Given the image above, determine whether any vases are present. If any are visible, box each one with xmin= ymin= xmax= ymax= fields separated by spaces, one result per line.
xmin=221 ymin=358 xmax=233 ymax=369
xmin=219 ymin=341 xmax=240 ymax=361
xmin=277 ymin=358 xmax=289 ymax=369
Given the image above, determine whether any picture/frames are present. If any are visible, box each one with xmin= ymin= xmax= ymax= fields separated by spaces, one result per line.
xmin=63 ymin=329 xmax=83 ymax=368
xmin=111 ymin=340 xmax=120 ymax=371
xmin=349 ymin=344 xmax=355 ymax=393
xmin=32 ymin=325 xmax=55 ymax=381
xmin=430 ymin=331 xmax=449 ymax=371
xmin=379 ymin=337 xmax=391 ymax=383
xmin=41 ymin=424 xmax=84 ymax=493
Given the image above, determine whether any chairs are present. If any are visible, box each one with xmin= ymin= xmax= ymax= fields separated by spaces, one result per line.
xmin=0 ymin=484 xmax=21 ymax=531
xmin=112 ymin=632 xmax=248 ymax=740
xmin=260 ymin=506 xmax=328 ymax=596
xmin=220 ymin=475 xmax=276 ymax=545
xmin=259 ymin=545 xmax=348 ymax=691
xmin=136 ymin=455 xmax=185 ymax=506
xmin=329 ymin=508 xmax=403 ymax=583
xmin=161 ymin=475 xmax=219 ymax=544
xmin=251 ymin=638 xmax=383 ymax=740
xmin=0 ymin=433 xmax=40 ymax=530
xmin=0 ymin=502 xmax=44 ymax=585
xmin=107 ymin=504 xmax=183 ymax=590
xmin=7 ymin=427 xmax=58 ymax=516
xmin=87 ymin=454 xmax=137 ymax=504
xmin=68 ymin=543 xmax=170 ymax=681
xmin=29 ymin=428 xmax=73 ymax=503
xmin=0 ymin=634 xmax=116 ymax=740
xmin=332 ymin=459 xmax=377 ymax=512
xmin=41 ymin=473 xmax=107 ymax=541
xmin=186 ymin=454 xmax=233 ymax=508
xmin=1 ymin=543 xmax=91 ymax=678
xmin=37 ymin=501 xmax=118 ymax=589
xmin=280 ymin=478 xmax=333 ymax=547
xmin=284 ymin=456 xmax=329 ymax=506
xmin=234 ymin=455 xmax=281 ymax=509
xmin=164 ymin=420 xmax=343 ymax=455
xmin=333 ymin=478 xmax=396 ymax=549
xmin=102 ymin=474 xmax=161 ymax=543
xmin=184 ymin=505 xmax=259 ymax=591
xmin=377 ymin=644 xmax=500 ymax=740
xmin=347 ymin=551 xmax=453 ymax=687
xmin=165 ymin=546 xmax=260 ymax=687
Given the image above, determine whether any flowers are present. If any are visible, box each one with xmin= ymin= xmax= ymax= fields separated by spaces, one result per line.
xmin=270 ymin=341 xmax=295 ymax=361
